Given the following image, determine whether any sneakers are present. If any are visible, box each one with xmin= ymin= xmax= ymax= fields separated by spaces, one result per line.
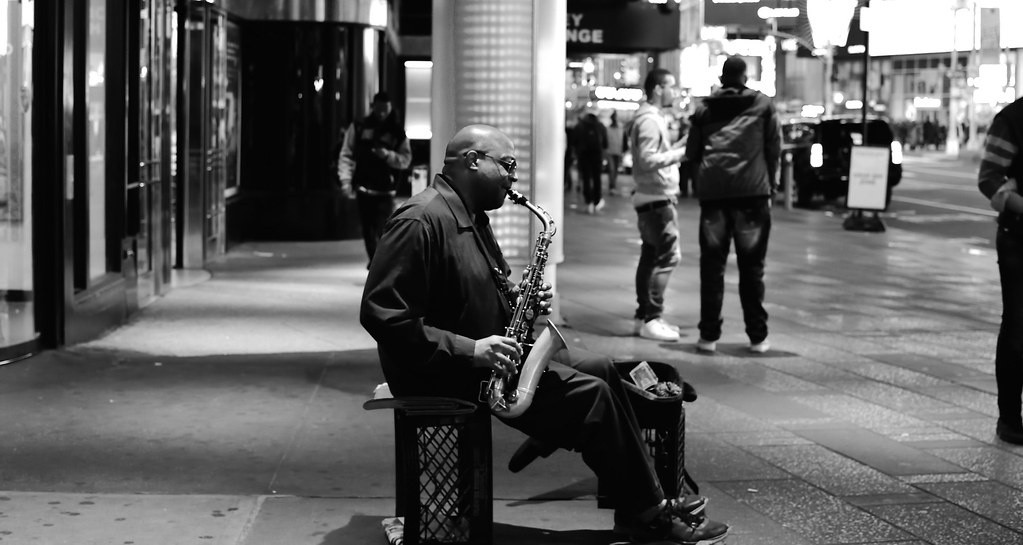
xmin=639 ymin=319 xmax=680 ymax=341
xmin=614 ymin=494 xmax=706 ymax=534
xmin=632 ymin=316 xmax=680 ymax=334
xmin=635 ymin=506 xmax=727 ymax=545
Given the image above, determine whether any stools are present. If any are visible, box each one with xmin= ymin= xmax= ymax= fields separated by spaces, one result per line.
xmin=363 ymin=389 xmax=493 ymax=544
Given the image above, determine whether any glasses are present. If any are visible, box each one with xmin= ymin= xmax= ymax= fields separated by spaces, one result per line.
xmin=462 ymin=148 xmax=516 ymax=172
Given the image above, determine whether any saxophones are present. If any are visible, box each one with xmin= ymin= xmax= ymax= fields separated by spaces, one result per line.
xmin=471 ymin=188 xmax=571 ymax=419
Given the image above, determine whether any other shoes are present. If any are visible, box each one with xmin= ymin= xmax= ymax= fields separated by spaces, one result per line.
xmin=585 ymin=201 xmax=593 ymax=213
xmin=608 ymin=187 xmax=615 ymax=195
xmin=751 ymin=335 xmax=770 ymax=352
xmin=996 ymin=417 xmax=1022 ymax=446
xmin=696 ymin=336 xmax=717 ymax=351
xmin=595 ymin=198 xmax=606 ymax=212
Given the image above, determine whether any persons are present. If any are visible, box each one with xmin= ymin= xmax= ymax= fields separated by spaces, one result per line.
xmin=893 ymin=118 xmax=965 ymax=150
xmin=337 ymin=93 xmax=411 ymax=269
xmin=626 ymin=70 xmax=687 ymax=343
xmin=358 ymin=123 xmax=730 ymax=545
xmin=978 ymin=93 xmax=1023 ymax=448
xmin=567 ymin=107 xmax=624 ymax=212
xmin=684 ymin=56 xmax=781 ymax=356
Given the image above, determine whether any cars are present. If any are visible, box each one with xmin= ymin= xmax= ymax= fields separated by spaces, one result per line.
xmin=780 ymin=113 xmax=903 ymax=210
